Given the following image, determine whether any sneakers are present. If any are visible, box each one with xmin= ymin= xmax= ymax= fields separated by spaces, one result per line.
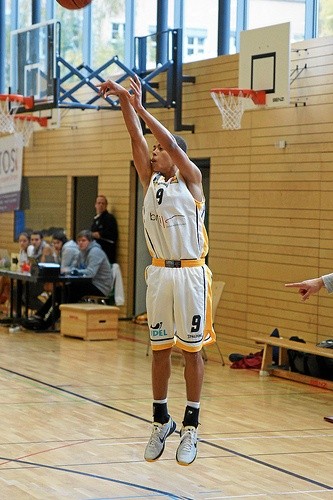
xmin=175 ymin=423 xmax=201 ymax=466
xmin=144 ymin=415 xmax=176 ymax=461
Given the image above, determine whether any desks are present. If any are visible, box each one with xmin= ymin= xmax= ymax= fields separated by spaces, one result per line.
xmin=0 ymin=267 xmax=92 ymax=333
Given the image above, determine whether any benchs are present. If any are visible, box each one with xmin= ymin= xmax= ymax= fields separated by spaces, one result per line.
xmin=250 ymin=336 xmax=333 ymax=391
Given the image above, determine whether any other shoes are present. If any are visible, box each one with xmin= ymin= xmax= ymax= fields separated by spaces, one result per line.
xmin=324 ymin=416 xmax=333 ymax=423
xmin=19 ymin=317 xmax=44 ymax=329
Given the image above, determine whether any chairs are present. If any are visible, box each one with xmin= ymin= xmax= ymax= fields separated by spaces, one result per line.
xmin=146 ymin=280 xmax=225 ymax=366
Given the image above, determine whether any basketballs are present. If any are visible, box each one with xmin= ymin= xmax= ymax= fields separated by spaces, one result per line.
xmin=55 ymin=0 xmax=92 ymax=10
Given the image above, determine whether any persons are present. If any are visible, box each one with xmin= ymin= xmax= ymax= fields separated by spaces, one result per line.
xmin=13 ymin=227 xmax=113 ymax=330
xmin=91 ymin=195 xmax=119 ymax=305
xmin=284 ymin=273 xmax=333 ymax=301
xmin=95 ymin=73 xmax=211 ymax=466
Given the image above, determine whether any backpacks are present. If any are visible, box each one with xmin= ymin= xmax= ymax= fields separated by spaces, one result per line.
xmin=287 ymin=335 xmax=333 ymax=381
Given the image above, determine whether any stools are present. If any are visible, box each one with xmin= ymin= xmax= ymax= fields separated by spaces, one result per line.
xmin=59 ymin=303 xmax=120 ymax=341
